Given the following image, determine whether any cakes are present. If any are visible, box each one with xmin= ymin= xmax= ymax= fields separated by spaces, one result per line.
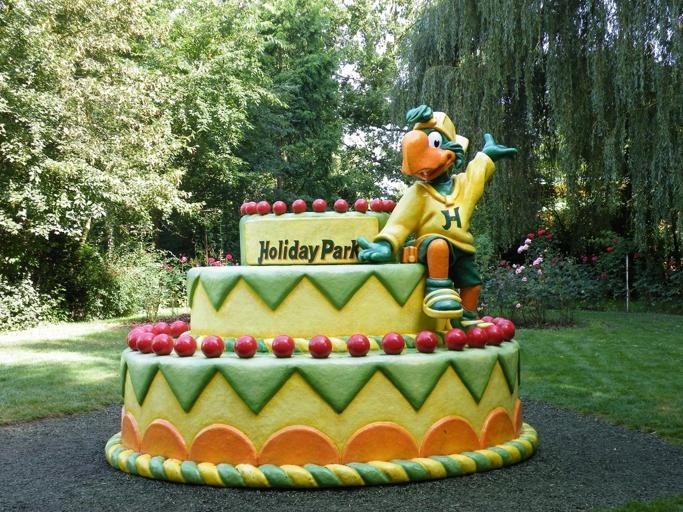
xmin=105 ymin=103 xmax=538 ymax=487
